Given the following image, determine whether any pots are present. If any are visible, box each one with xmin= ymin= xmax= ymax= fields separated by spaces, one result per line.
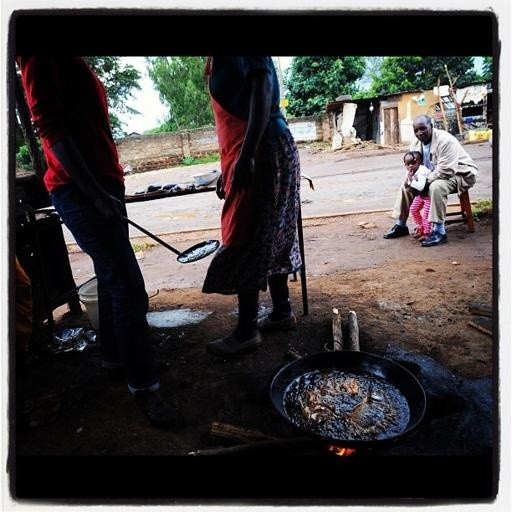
xmin=270 ymin=350 xmax=428 ymax=447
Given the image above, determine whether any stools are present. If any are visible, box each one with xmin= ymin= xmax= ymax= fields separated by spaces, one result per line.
xmin=446 ymin=190 xmax=476 ymax=234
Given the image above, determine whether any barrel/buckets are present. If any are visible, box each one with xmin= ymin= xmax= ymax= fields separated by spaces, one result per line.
xmin=76 ymin=276 xmax=100 ymax=330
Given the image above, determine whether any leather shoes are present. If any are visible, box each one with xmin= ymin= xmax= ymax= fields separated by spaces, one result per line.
xmin=383 ymin=224 xmax=409 ymax=239
xmin=422 ymin=230 xmax=448 ymax=247
xmin=205 ymin=328 xmax=261 ymax=357
xmin=253 ymin=311 xmax=298 ymax=329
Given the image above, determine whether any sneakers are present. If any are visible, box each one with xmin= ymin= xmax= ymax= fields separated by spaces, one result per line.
xmin=100 ymin=349 xmax=125 ymax=369
xmin=413 ymin=228 xmax=430 ymax=241
xmin=127 ymin=348 xmax=159 ymax=395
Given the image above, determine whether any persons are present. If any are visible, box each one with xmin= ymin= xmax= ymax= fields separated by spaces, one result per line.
xmin=12 ymin=41 xmax=165 ymax=394
xmin=383 ymin=114 xmax=477 ymax=245
xmin=205 ymin=50 xmax=305 ymax=353
